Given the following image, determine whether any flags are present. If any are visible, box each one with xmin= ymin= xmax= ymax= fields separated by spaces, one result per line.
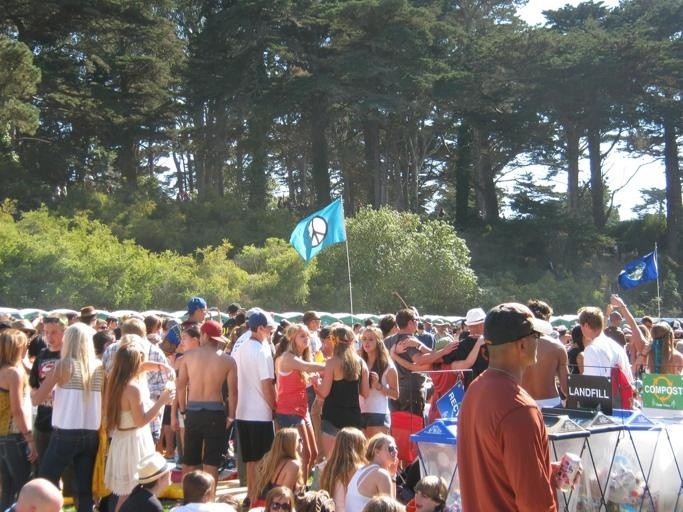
xmin=290 ymin=196 xmax=347 ymax=262
xmin=617 ymin=250 xmax=659 ymax=291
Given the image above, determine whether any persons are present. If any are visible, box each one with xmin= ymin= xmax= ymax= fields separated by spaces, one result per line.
xmin=0 ymin=296 xmax=683 ymax=512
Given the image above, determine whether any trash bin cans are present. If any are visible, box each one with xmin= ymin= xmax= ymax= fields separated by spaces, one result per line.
xmin=409 ymin=408 xmax=683 ymax=512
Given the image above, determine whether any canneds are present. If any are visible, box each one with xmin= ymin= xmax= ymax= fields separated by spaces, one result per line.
xmin=556 ymin=453 xmax=580 ymax=492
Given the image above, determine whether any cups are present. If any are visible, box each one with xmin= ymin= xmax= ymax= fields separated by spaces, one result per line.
xmin=610 ymin=293 xmax=619 ymax=308
xmin=313 ymin=350 xmax=324 ymax=364
xmin=164 ymin=381 xmax=176 ymax=406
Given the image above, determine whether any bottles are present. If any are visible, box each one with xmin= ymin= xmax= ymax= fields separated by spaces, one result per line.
xmin=621 ymin=470 xmax=650 ymax=512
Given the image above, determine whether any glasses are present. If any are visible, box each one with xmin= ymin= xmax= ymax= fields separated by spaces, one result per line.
xmin=270 ymin=502 xmax=291 ymax=510
xmin=381 ymin=446 xmax=398 ymax=452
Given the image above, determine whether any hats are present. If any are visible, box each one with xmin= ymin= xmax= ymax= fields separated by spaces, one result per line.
xmin=245 ymin=307 xmax=281 ymax=328
xmin=77 ymin=305 xmax=98 ymax=319
xmin=184 ymin=297 xmax=231 ymax=342
xmin=133 ymin=451 xmax=177 ymax=485
xmin=466 ymin=302 xmax=553 ymax=346
xmin=12 ymin=318 xmax=35 ymax=335
